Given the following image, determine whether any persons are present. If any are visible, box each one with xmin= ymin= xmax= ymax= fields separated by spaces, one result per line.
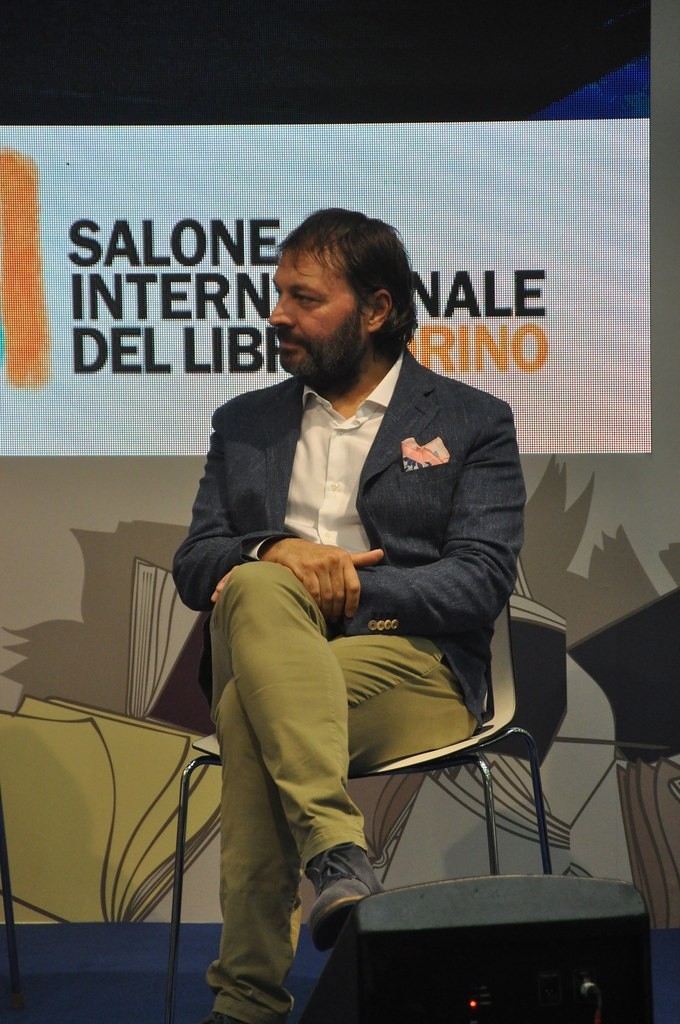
xmin=170 ymin=205 xmax=527 ymax=1022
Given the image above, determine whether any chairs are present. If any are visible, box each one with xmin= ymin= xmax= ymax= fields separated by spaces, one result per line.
xmin=162 ymin=597 xmax=554 ymax=1024
